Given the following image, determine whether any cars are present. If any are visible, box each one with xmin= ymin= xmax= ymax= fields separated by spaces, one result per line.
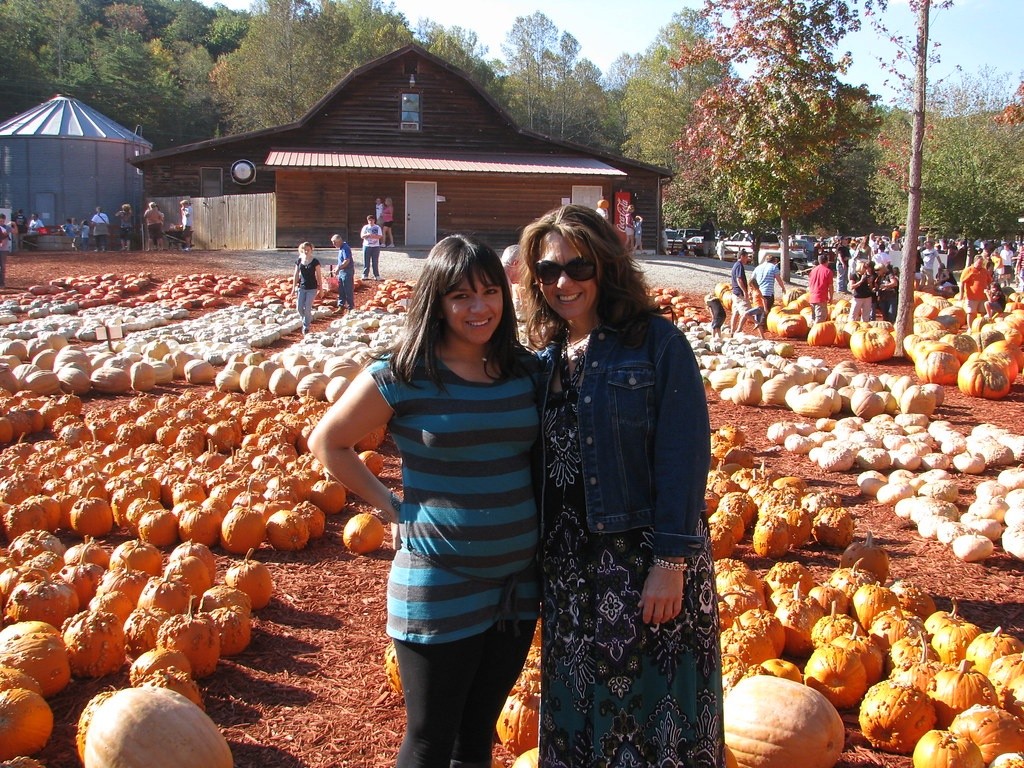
xmin=665 ymin=228 xmax=821 ymax=266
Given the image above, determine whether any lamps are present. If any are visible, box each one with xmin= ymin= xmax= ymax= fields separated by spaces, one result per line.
xmin=409 ymin=74 xmax=416 ymax=88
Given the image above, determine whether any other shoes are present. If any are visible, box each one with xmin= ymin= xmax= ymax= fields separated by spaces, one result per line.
xmin=183 ymin=247 xmax=192 ymax=252
xmin=387 ymin=243 xmax=395 ymax=248
xmin=380 ymin=243 xmax=387 ymax=248
xmin=374 ymin=275 xmax=381 ymax=281
xmin=302 ymin=328 xmax=309 ymax=336
xmin=333 ymin=306 xmax=345 ymax=315
xmin=361 ymin=275 xmax=368 ymax=281
xmin=752 ymin=322 xmax=760 ymax=330
xmin=838 ymin=290 xmax=853 ymax=295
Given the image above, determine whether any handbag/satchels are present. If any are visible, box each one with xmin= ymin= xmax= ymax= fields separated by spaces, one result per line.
xmin=38 ymin=227 xmax=49 ymax=235
xmin=877 ymin=288 xmax=895 ymax=302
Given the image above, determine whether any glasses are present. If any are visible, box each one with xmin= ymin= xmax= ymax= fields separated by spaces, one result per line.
xmin=533 ymin=256 xmax=598 ymax=285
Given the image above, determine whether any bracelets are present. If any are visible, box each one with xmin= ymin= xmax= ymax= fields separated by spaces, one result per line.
xmin=372 ymin=490 xmax=401 ymax=523
xmin=652 ymin=555 xmax=688 ymax=572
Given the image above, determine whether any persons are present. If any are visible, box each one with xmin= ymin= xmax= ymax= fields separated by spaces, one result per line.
xmin=331 ymin=234 xmax=354 ymax=313
xmin=179 ymin=200 xmax=193 ymax=252
xmin=595 ymin=199 xmax=608 ymax=220
xmin=115 ymin=204 xmax=133 ymax=250
xmin=91 ymin=207 xmax=110 ymax=252
xmin=291 ymin=242 xmax=324 ymax=334
xmin=625 ymin=204 xmax=635 ymax=254
xmin=706 ymin=226 xmax=1023 ymax=347
xmin=143 ymin=201 xmax=164 ymax=251
xmin=520 ymin=204 xmax=726 ymax=768
xmin=376 ymin=198 xmax=384 ymax=226
xmin=633 ymin=215 xmax=648 ymax=255
xmin=0 ymin=201 xmax=91 ymax=286
xmin=360 ymin=215 xmax=385 ymax=281
xmin=381 ymin=197 xmax=395 ymax=248
xmin=305 ymin=232 xmax=552 ymax=768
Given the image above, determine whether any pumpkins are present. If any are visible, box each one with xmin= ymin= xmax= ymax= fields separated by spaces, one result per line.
xmin=642 ymin=280 xmax=1024 ymax=768
xmin=0 ymin=273 xmax=540 ymax=768
